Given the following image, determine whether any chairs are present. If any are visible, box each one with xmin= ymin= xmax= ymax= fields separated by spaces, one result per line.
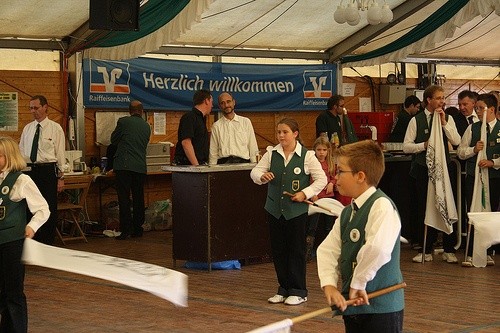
xmin=57 ymin=174 xmax=91 ymax=245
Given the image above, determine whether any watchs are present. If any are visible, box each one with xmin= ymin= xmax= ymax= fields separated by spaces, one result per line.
xmin=57 ymin=176 xmax=64 ymax=180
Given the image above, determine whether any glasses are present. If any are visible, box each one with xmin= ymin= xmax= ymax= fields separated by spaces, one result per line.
xmin=337 ymin=169 xmax=366 ymax=181
xmin=474 ymin=104 xmax=494 ymax=112
xmin=430 ymin=96 xmax=445 ymax=100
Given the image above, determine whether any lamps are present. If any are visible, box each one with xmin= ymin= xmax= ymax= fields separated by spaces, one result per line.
xmin=333 ymin=0 xmax=393 ymax=26
xmin=386 ymin=73 xmax=404 ymax=85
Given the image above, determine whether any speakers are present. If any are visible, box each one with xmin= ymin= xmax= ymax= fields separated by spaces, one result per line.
xmin=89 ymin=0 xmax=139 ymax=31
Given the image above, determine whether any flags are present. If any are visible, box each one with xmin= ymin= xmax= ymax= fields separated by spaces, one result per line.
xmin=424 ymin=111 xmax=458 ymax=235
xmin=469 ymin=123 xmax=491 ymax=268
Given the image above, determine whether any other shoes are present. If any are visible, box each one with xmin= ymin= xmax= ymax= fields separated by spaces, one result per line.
xmin=467 ymin=256 xmax=472 ymax=262
xmin=116 ymin=227 xmax=144 ymax=240
xmin=487 ymin=252 xmax=495 ymax=266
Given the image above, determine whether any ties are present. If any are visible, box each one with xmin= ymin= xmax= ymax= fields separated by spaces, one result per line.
xmin=30 ymin=123 xmax=41 ymax=163
xmin=467 ymin=115 xmax=473 ymax=124
xmin=486 ymin=123 xmax=490 ymax=134
xmin=430 ymin=114 xmax=434 ymax=130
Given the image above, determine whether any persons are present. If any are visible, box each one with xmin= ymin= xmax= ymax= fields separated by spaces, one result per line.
xmin=458 ymin=90 xmax=500 ymax=267
xmin=19 ymin=95 xmax=65 ymax=246
xmin=317 ymin=141 xmax=405 ymax=333
xmin=250 ymin=117 xmax=328 ymax=306
xmin=111 ymin=100 xmax=151 ymax=240
xmin=387 ymin=95 xmax=422 ymax=156
xmin=209 ymin=92 xmax=260 ymax=166
xmin=175 ymin=89 xmax=213 ymax=165
xmin=0 ymin=137 xmax=51 ymax=333
xmin=304 ymin=136 xmax=337 ymax=263
xmin=403 ymin=86 xmax=461 ymax=263
xmin=316 ymin=95 xmax=345 ymax=148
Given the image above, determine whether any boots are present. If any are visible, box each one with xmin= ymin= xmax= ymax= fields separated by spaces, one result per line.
xmin=306 ymin=235 xmax=315 ymax=264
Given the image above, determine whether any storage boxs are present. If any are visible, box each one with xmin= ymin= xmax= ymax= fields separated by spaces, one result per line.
xmin=379 ymin=85 xmax=406 ymax=104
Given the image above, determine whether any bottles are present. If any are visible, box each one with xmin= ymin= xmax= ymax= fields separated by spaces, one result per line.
xmin=101 ymin=156 xmax=108 ymax=172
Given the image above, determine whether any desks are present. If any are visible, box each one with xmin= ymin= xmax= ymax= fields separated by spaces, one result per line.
xmin=99 ymin=170 xmax=172 ymax=234
xmin=162 ymin=150 xmax=462 ymax=272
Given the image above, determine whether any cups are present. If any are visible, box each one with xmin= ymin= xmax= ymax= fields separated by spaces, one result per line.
xmin=73 ymin=162 xmax=85 ymax=172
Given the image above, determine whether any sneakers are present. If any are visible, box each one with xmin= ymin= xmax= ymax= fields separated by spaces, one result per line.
xmin=266 ymin=294 xmax=287 ymax=303
xmin=284 ymin=296 xmax=307 ymax=305
xmin=412 ymin=253 xmax=433 ymax=263
xmin=441 ymin=252 xmax=458 ymax=262
